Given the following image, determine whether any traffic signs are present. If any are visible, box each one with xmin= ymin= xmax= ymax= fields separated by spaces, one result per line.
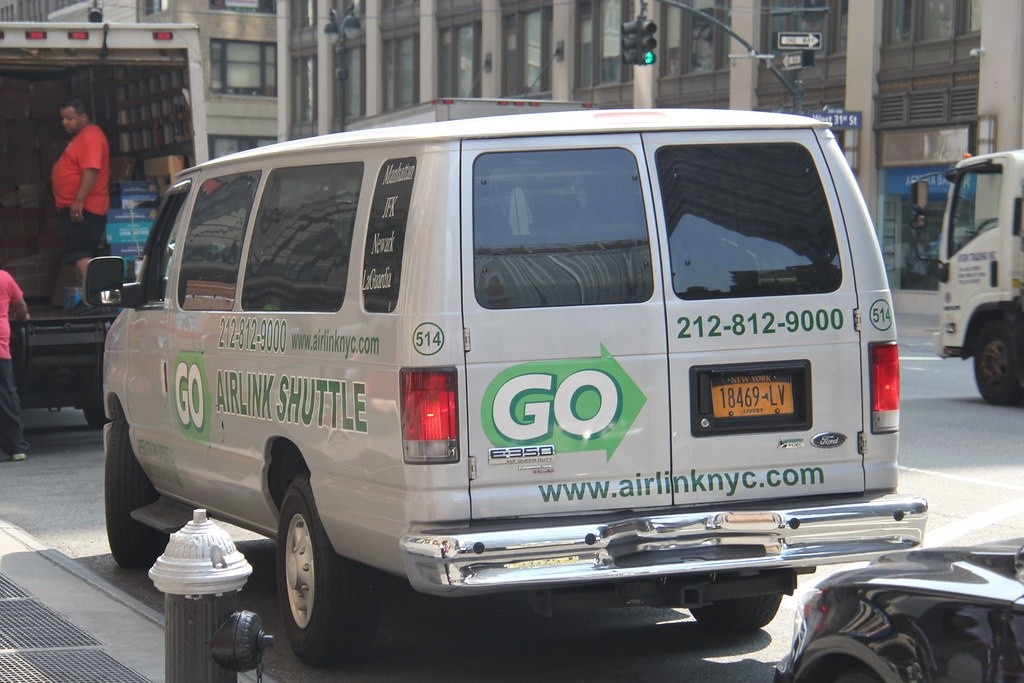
xmin=780 ymin=54 xmax=803 ymax=70
xmin=809 ymin=112 xmax=862 ymax=130
xmin=778 ymin=32 xmax=823 ymax=50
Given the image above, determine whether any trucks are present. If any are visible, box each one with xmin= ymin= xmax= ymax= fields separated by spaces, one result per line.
xmin=904 ymin=149 xmax=1022 ymax=410
xmin=0 ymin=23 xmax=209 ymax=432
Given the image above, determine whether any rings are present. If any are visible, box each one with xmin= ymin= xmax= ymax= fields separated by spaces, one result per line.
xmin=75 ymin=214 xmax=78 ymax=216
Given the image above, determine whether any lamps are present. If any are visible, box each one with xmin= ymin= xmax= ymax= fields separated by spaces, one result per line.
xmin=970 ymin=47 xmax=987 ymax=58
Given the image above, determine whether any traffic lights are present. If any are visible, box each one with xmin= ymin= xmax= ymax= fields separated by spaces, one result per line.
xmin=620 ymin=20 xmax=659 ymax=66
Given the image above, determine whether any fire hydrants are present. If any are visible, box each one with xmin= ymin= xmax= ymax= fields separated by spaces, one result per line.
xmin=142 ymin=509 xmax=276 ymax=683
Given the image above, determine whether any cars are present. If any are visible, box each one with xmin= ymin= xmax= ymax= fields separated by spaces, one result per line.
xmin=770 ymin=535 xmax=1024 ymax=683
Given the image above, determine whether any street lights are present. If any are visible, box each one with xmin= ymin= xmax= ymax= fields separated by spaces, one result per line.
xmin=323 ymin=4 xmax=362 ymax=134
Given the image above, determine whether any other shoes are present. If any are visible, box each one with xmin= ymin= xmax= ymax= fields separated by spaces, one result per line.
xmin=71 ymin=301 xmax=105 ymax=316
xmin=10 ymin=447 xmax=27 ymax=461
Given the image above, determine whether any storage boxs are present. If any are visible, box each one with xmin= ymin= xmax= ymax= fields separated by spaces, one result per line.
xmin=144 ymin=155 xmax=183 ymax=177
xmin=157 ymin=173 xmax=176 ymax=193
xmin=0 ymin=77 xmax=81 ymax=308
xmin=96 ymin=180 xmax=157 ymax=280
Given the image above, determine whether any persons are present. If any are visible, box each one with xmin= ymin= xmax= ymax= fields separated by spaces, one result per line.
xmin=0 ymin=268 xmax=30 ymax=461
xmin=51 ymin=100 xmax=109 ymax=307
xmin=905 ymin=232 xmax=930 ymax=290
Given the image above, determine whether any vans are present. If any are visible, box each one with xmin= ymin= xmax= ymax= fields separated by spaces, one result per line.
xmin=82 ymin=110 xmax=929 ymax=669
